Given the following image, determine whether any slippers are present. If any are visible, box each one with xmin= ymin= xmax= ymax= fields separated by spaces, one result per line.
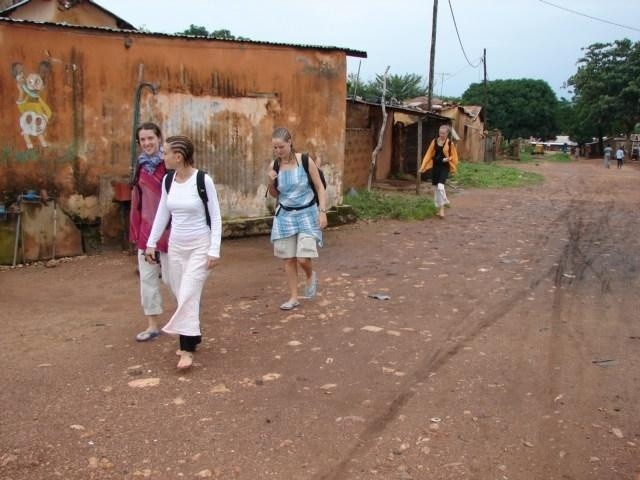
xmin=279 ymin=300 xmax=301 ymax=310
xmin=304 ymin=270 xmax=316 ymax=299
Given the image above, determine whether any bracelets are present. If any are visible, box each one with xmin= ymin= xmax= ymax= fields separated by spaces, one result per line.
xmin=319 ymin=208 xmax=327 ymax=213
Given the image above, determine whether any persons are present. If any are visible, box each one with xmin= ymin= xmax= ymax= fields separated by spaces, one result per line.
xmin=602 ymin=144 xmax=613 ymax=169
xmin=265 ymin=127 xmax=328 ymax=310
xmin=129 ymin=122 xmax=175 ymax=342
xmin=144 ymin=134 xmax=222 ymax=371
xmin=562 ymin=143 xmax=568 ymax=153
xmin=614 ymin=147 xmax=625 ymax=169
xmin=418 ymin=124 xmax=458 ymax=217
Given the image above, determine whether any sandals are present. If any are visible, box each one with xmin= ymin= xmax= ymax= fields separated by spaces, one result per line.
xmin=136 ymin=328 xmax=161 ymax=342
xmin=175 ymin=349 xmax=193 ymax=370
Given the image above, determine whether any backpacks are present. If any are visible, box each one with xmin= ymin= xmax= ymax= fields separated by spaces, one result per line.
xmin=273 ymin=152 xmax=327 ymax=210
